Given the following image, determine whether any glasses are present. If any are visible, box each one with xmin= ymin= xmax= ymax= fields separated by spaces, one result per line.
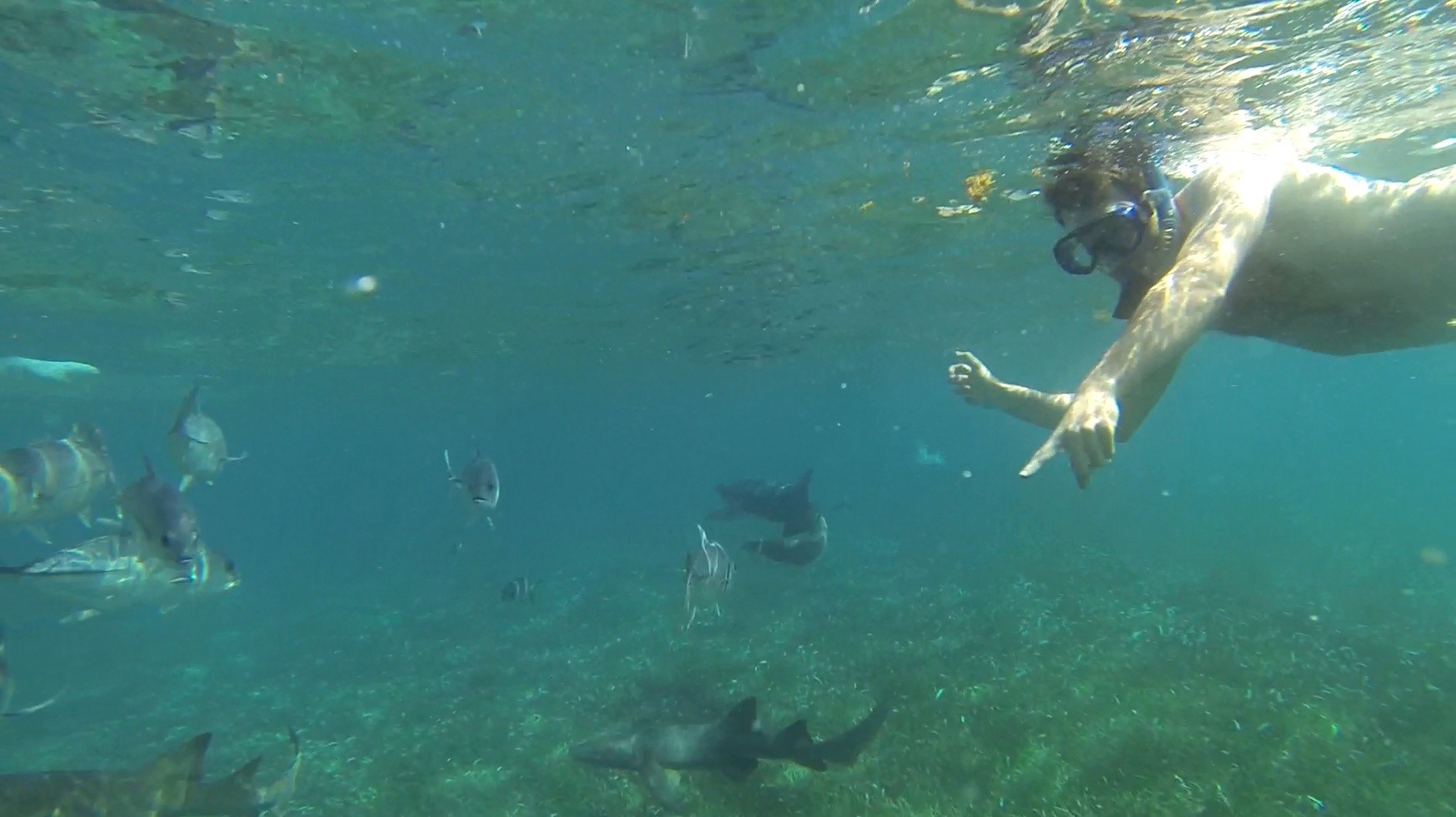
xmin=1052 ymin=201 xmax=1153 ymax=275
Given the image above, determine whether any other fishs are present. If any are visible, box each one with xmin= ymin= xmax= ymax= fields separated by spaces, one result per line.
xmin=0 ymin=2 xmax=1454 ymax=817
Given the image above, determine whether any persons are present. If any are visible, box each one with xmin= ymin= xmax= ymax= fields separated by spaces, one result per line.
xmin=946 ymin=124 xmax=1455 ymax=491
